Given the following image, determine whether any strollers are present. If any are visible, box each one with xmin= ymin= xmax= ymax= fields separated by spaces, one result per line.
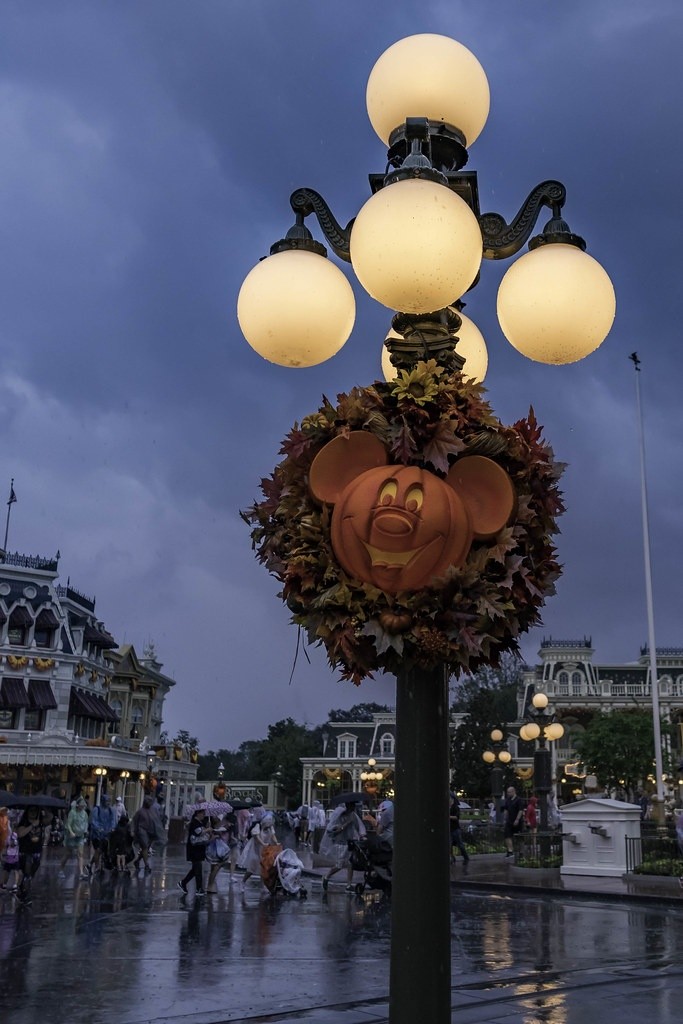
xmin=346 ymin=832 xmax=394 ymax=898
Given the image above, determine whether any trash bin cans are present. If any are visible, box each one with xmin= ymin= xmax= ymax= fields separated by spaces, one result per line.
xmin=168 ymin=816 xmax=190 ymax=843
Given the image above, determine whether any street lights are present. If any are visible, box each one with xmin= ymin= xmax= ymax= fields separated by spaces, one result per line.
xmin=482 ymin=729 xmax=513 ymax=851
xmin=518 ymin=691 xmax=565 ymax=868
xmin=144 ymin=747 xmax=158 ymax=795
xmin=231 ymin=29 xmax=618 ymax=1024
xmin=217 ymin=761 xmax=228 ymax=785
xmin=359 ymin=757 xmax=384 ymax=832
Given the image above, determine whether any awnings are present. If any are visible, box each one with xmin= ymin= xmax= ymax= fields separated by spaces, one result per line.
xmin=36 ymin=608 xmax=59 ymax=629
xmin=0 ymin=606 xmax=7 ymax=627
xmin=27 ymin=679 xmax=57 ymax=711
xmin=0 ymin=677 xmax=30 ymax=708
xmin=70 ymin=686 xmax=120 ymax=723
xmin=9 ymin=605 xmax=34 ymax=629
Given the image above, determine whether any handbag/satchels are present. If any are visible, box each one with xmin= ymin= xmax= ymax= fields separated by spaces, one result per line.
xmin=190 ymin=820 xmax=210 ymax=844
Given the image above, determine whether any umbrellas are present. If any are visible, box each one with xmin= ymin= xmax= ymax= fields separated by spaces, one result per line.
xmin=378 ymin=801 xmax=394 ymax=810
xmin=332 ymin=793 xmax=370 ymax=817
xmin=0 ymin=791 xmax=68 ymax=831
xmin=450 ymin=793 xmax=471 ymax=809
xmin=183 ymin=799 xmax=262 ymax=835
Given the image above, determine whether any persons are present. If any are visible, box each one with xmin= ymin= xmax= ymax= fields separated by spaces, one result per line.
xmin=0 ymin=807 xmax=53 ymax=907
xmin=319 ymin=802 xmax=371 ymax=890
xmin=377 ymin=806 xmax=394 ymax=850
xmin=500 ymin=786 xmax=542 ymax=858
xmin=177 ymin=807 xmax=277 ymax=897
xmin=286 ymin=800 xmax=326 ymax=854
xmin=56 ymin=795 xmax=170 ymax=879
xmin=449 ymin=797 xmax=470 ymax=865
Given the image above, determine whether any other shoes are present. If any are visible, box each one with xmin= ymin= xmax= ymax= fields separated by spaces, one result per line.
xmin=240 ymin=880 xmax=245 ymax=893
xmin=462 ymin=857 xmax=471 ymax=865
xmin=261 ymin=887 xmax=270 ymax=894
xmin=322 ymin=875 xmax=328 ymax=891
xmin=58 ymin=872 xmax=66 ymax=879
xmin=117 ymin=864 xmax=129 ymax=871
xmin=230 ymin=877 xmax=237 ymax=883
xmin=345 ymin=886 xmax=355 ymax=893
xmin=144 ymin=864 xmax=151 ymax=872
xmin=85 ymin=864 xmax=94 ymax=875
xmin=79 ymin=873 xmax=90 ymax=880
xmin=9 ymin=888 xmax=18 ymax=893
xmin=0 ymin=884 xmax=7 ymax=891
xmin=15 ymin=894 xmax=32 ymax=905
xmin=196 ymin=890 xmax=206 ymax=895
xmin=177 ymin=881 xmax=188 ymax=894
xmin=134 ymin=860 xmax=140 ymax=870
